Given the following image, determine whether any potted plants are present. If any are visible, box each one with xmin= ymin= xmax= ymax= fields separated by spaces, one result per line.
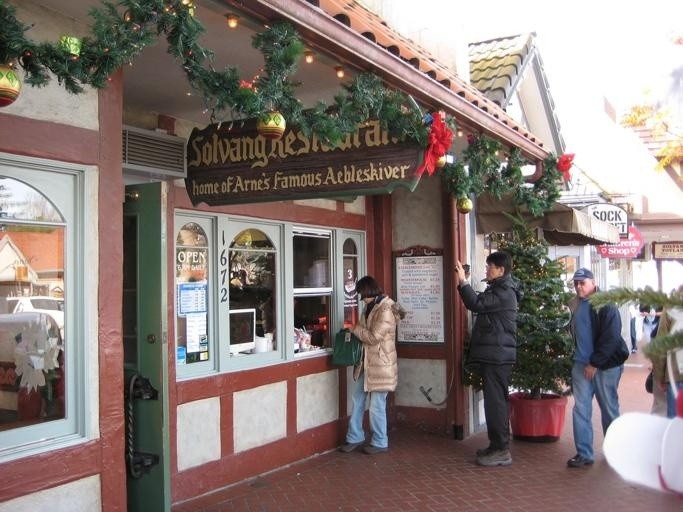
xmin=461 ymin=202 xmax=577 ymax=444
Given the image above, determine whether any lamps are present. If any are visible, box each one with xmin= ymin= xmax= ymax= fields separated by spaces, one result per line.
xmin=303 ymin=46 xmax=313 ymax=64
xmin=333 ymin=62 xmax=345 ymax=78
xmin=456 ymin=127 xmax=464 ymax=137
xmin=222 ymin=8 xmax=238 ymax=29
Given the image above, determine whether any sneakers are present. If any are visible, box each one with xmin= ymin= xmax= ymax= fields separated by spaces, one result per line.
xmin=339 ymin=441 xmax=365 ymax=452
xmin=477 ymin=447 xmax=495 ymax=455
xmin=567 ymin=454 xmax=593 ymax=467
xmin=476 ymin=450 xmax=512 ymax=466
xmin=362 ymin=443 xmax=389 ymax=455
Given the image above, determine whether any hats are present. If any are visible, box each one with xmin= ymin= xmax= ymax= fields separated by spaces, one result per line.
xmin=572 ymin=267 xmax=593 ymax=281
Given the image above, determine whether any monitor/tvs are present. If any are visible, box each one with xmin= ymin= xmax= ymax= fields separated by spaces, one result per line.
xmin=229 ymin=308 xmax=257 ymax=354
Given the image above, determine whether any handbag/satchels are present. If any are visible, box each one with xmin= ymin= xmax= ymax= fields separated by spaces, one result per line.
xmin=331 ymin=329 xmax=362 ymax=366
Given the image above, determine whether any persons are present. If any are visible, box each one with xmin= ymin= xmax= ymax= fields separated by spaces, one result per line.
xmin=337 ymin=275 xmax=407 ymax=456
xmin=649 ymin=359 xmax=666 ymax=418
xmin=566 ymin=267 xmax=629 ymax=469
xmin=630 ymin=301 xmax=652 ymax=353
xmin=292 ymin=297 xmax=320 ymax=328
xmin=651 ymin=285 xmax=683 ymax=420
xmin=454 ymin=251 xmax=520 ymax=469
xmin=649 ymin=304 xmax=672 ymax=338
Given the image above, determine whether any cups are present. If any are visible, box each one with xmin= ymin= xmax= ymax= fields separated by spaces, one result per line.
xmin=301 ymin=334 xmax=311 ymax=350
xmin=264 ymin=333 xmax=274 ymax=351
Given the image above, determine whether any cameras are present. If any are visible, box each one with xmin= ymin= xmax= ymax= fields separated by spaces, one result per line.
xmin=454 ymin=264 xmax=470 ymax=275
